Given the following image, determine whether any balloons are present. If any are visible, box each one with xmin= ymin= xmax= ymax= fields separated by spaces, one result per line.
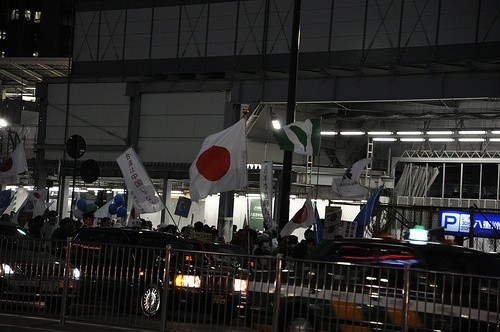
xmin=108 ymin=195 xmax=127 ymax=217
xmin=75 ymin=200 xmax=97 ymax=220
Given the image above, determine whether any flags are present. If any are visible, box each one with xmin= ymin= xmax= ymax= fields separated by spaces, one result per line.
xmin=0 ymin=142 xmax=27 ymax=179
xmin=281 ymin=193 xmax=323 ymax=245
xmin=354 ymin=186 xmax=383 ymax=226
xmin=3 ymin=187 xmax=52 ymax=229
xmin=218 ymin=220 xmax=232 ymax=242
xmin=189 ymin=117 xmax=246 ymax=204
xmin=331 ymin=157 xmax=372 ymax=196
xmin=273 ymin=117 xmax=321 ymax=156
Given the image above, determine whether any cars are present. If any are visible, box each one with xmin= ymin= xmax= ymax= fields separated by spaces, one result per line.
xmin=62 ymin=226 xmax=249 ymax=321
xmin=246 ymin=236 xmax=500 ymax=332
xmin=0 ymin=220 xmax=81 ymax=315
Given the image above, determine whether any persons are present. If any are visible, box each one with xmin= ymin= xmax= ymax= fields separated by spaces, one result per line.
xmin=0 ymin=210 xmax=323 ymax=286
